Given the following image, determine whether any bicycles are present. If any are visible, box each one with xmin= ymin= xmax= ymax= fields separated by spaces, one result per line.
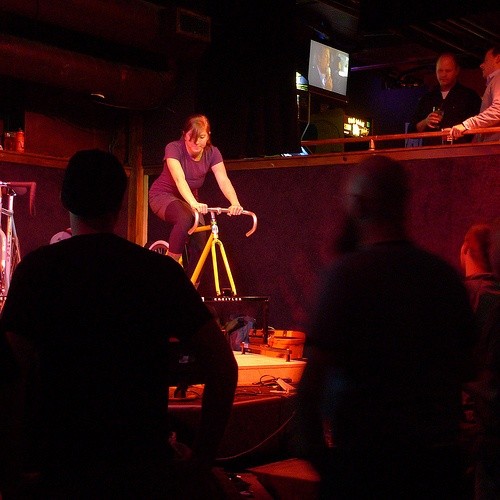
xmin=143 ymin=207 xmax=258 ymax=297
xmin=0 ymin=181 xmax=37 ymax=311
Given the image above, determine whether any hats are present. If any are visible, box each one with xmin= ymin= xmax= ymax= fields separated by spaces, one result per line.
xmin=61 ymin=148 xmax=128 ymax=217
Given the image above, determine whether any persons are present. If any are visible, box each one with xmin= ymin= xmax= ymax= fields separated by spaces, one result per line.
xmin=148 ymin=113 xmax=243 ymax=262
xmin=451 ymin=44 xmax=500 ymax=143
xmin=310 ymin=46 xmax=336 ymax=92
xmin=460 ymin=221 xmax=500 ymax=500
xmin=294 ymin=158 xmax=474 ymax=500
xmin=406 ymin=50 xmax=483 ymax=145
xmin=0 ymin=147 xmax=243 ymax=500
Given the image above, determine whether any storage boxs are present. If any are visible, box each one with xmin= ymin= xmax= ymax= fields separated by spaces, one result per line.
xmin=249 ymin=329 xmax=305 ymax=360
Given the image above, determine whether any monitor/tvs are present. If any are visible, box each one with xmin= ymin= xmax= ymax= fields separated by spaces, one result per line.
xmin=307 ymin=40 xmax=350 ymax=103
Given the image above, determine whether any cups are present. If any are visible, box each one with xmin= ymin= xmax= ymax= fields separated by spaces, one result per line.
xmin=432 ymin=107 xmax=440 ymax=125
xmin=442 ymin=128 xmax=453 ymax=144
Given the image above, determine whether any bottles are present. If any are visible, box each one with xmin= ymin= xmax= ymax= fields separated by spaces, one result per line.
xmin=16 ymin=128 xmax=24 ymax=152
